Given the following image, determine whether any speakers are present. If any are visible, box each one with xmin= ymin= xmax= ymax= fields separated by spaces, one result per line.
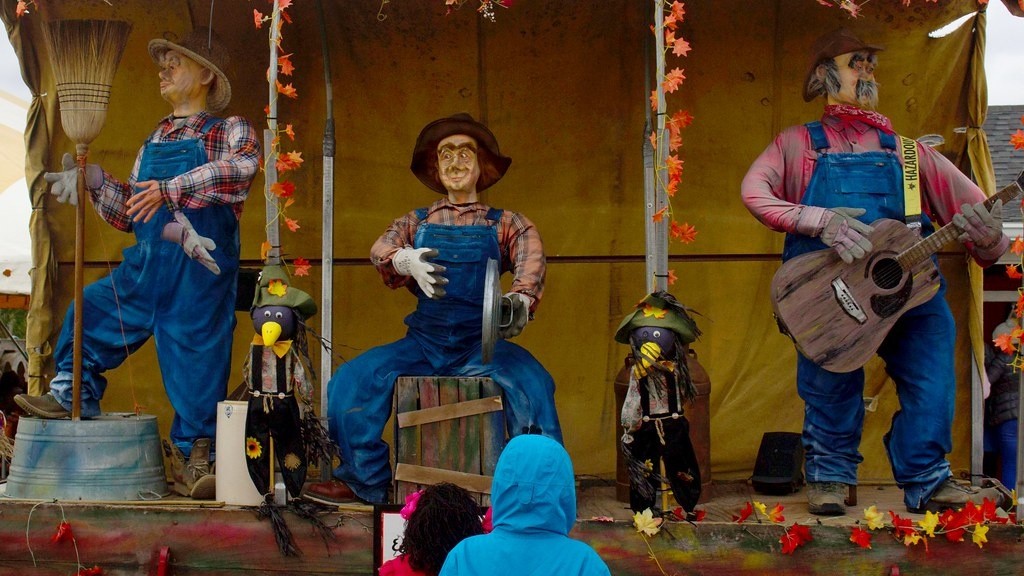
xmin=752 ymin=432 xmax=805 ymax=494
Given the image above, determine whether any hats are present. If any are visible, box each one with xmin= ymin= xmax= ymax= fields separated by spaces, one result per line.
xmin=801 ymin=26 xmax=884 ymax=103
xmin=409 ymin=113 xmax=512 ymax=195
xmin=148 ymin=25 xmax=231 ymax=112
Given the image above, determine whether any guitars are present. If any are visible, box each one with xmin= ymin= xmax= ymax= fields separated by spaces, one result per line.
xmin=771 ymin=170 xmax=1023 ymax=376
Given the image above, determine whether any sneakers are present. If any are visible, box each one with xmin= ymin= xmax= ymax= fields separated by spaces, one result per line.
xmin=162 ymin=436 xmax=215 ymax=499
xmin=805 ymin=480 xmax=850 ymax=515
xmin=906 ymin=474 xmax=1003 ymax=514
xmin=14 ymin=392 xmax=72 ymax=419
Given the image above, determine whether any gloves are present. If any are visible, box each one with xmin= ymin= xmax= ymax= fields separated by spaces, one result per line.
xmin=392 ymin=246 xmax=448 ymax=300
xmin=816 ymin=207 xmax=873 ymax=264
xmin=175 ymin=210 xmax=220 ymax=276
xmin=952 ymin=199 xmax=1003 ymax=248
xmin=497 ymin=291 xmax=530 ymax=340
xmin=43 ymin=153 xmax=103 ymax=207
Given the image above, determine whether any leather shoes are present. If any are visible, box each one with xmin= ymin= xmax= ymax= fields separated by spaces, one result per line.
xmin=305 ymin=478 xmax=358 ymax=507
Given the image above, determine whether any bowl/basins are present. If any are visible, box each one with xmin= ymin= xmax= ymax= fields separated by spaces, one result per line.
xmin=0 ymin=413 xmax=172 ymax=501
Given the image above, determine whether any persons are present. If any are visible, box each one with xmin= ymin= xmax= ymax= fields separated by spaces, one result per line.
xmin=0 ymin=360 xmax=26 ymax=482
xmin=12 ymin=26 xmax=259 ymax=500
xmin=437 ymin=433 xmax=611 ymax=576
xmin=301 ymin=112 xmax=564 ymax=507
xmin=613 ymin=291 xmax=702 ymax=522
xmin=739 ymin=24 xmax=1011 ymax=516
xmin=377 ymin=480 xmax=482 ymax=575
xmin=242 ymin=264 xmax=318 ymax=511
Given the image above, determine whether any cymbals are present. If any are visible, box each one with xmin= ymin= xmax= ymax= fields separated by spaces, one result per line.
xmin=479 ymin=256 xmax=516 ymax=366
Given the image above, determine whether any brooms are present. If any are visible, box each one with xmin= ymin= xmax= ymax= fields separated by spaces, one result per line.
xmin=37 ymin=17 xmax=136 ymax=421
